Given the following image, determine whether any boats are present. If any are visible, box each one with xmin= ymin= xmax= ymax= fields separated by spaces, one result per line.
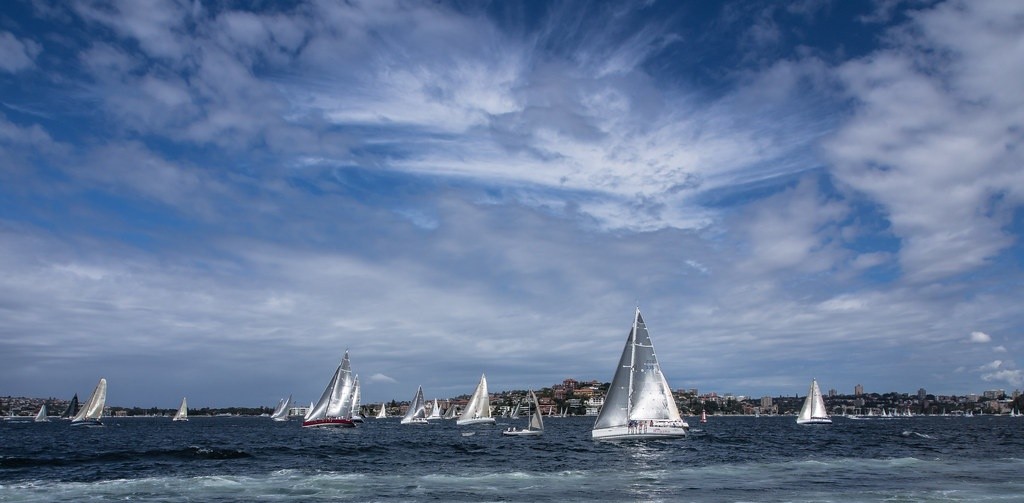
xmin=216 ymin=413 xmax=231 ymax=416
xmin=259 ymin=413 xmax=270 ymax=418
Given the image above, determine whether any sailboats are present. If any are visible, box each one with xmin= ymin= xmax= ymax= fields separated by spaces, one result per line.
xmin=301 ymin=348 xmax=357 ymax=428
xmin=591 ymin=306 xmax=689 ymax=439
xmin=796 ymin=379 xmax=833 ymax=425
xmin=867 ymin=408 xmax=912 ymax=417
xmin=401 ymin=385 xmax=429 ymax=424
xmin=456 ymin=372 xmax=496 ymax=425
xmin=502 ymin=385 xmax=544 ymax=436
xmin=269 ymin=348 xmax=569 ymax=437
xmin=60 ymin=392 xmax=79 ymax=420
xmin=34 ymin=404 xmax=52 ymax=422
xmin=173 ymin=397 xmax=189 ymax=422
xmin=70 ymin=377 xmax=107 ymax=427
